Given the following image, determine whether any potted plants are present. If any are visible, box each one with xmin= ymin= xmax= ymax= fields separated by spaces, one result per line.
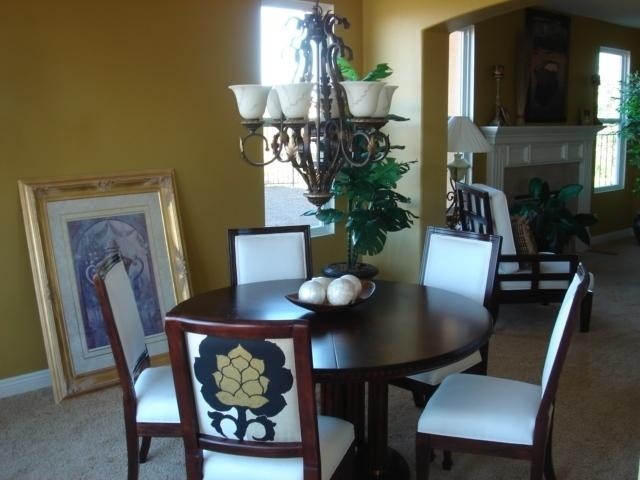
xmin=607 ymin=69 xmax=640 ymax=247
xmin=299 ymin=57 xmax=421 ymax=279
xmin=510 ymin=176 xmax=598 ymax=299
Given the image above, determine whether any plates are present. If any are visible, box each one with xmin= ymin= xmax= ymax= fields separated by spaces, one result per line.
xmin=283 ymin=280 xmax=377 ymax=316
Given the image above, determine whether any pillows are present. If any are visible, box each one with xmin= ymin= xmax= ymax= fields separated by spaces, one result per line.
xmin=512 ymin=214 xmax=543 ymax=269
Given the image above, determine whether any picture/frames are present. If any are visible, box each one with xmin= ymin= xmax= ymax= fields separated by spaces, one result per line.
xmin=579 ymin=106 xmax=593 ymax=125
xmin=523 ymin=7 xmax=571 ymax=125
xmin=15 ymin=165 xmax=198 ymax=404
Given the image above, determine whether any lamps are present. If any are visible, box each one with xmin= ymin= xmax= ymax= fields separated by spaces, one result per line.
xmin=227 ymin=3 xmax=400 ymax=216
xmin=444 ymin=115 xmax=493 ymax=213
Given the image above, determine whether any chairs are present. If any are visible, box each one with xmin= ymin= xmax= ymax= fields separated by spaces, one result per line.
xmin=226 ymin=224 xmax=314 ymax=287
xmin=366 ymin=225 xmax=504 ymax=472
xmin=163 ymin=312 xmax=361 ymax=479
xmin=93 ymin=250 xmax=185 ymax=479
xmin=414 ymin=260 xmax=589 ymax=479
xmin=453 ymin=181 xmax=597 ymax=333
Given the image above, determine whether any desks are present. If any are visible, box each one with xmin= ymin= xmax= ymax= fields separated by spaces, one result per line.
xmin=167 ymin=274 xmax=495 ymax=479
